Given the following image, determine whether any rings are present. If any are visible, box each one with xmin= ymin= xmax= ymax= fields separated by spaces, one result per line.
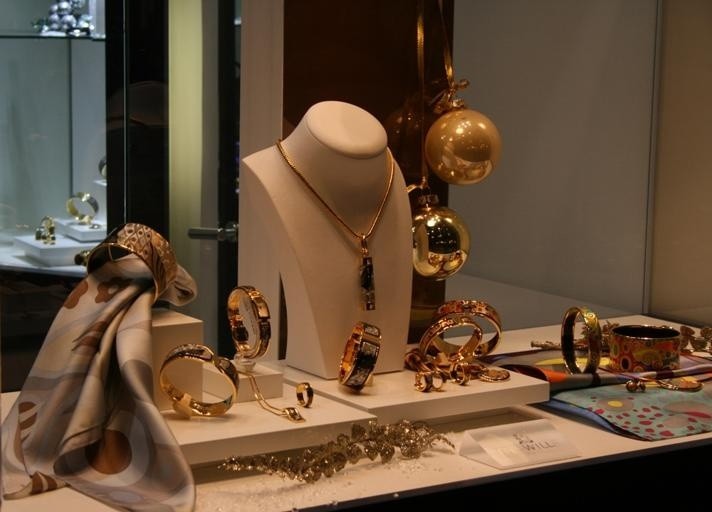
xmin=414 ymin=359 xmax=471 ymax=393
xmin=296 ymin=383 xmax=313 ymax=409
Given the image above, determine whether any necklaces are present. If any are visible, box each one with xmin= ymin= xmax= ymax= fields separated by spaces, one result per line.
xmin=238 ymin=369 xmax=308 ymax=424
xmin=277 ymin=136 xmax=396 ymax=313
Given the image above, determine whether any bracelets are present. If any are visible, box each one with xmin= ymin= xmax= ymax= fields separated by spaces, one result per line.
xmin=158 ymin=342 xmax=238 ymax=421
xmin=560 ymin=304 xmax=602 ymax=376
xmin=419 ymin=316 xmax=482 ymax=366
xmin=335 ymin=322 xmax=382 ymax=391
xmin=97 ymin=155 xmax=107 ymax=181
xmin=34 ymin=215 xmax=57 ymax=244
xmin=434 ymin=298 xmax=502 ymax=359
xmin=87 ymin=224 xmax=176 ymax=304
xmin=228 ymin=284 xmax=270 ymax=363
xmin=64 ymin=190 xmax=99 ymax=224
xmin=656 ymin=373 xmax=703 ymax=393
xmin=608 ymin=324 xmax=682 ymax=372
xmin=75 ymin=250 xmax=94 ymax=263
